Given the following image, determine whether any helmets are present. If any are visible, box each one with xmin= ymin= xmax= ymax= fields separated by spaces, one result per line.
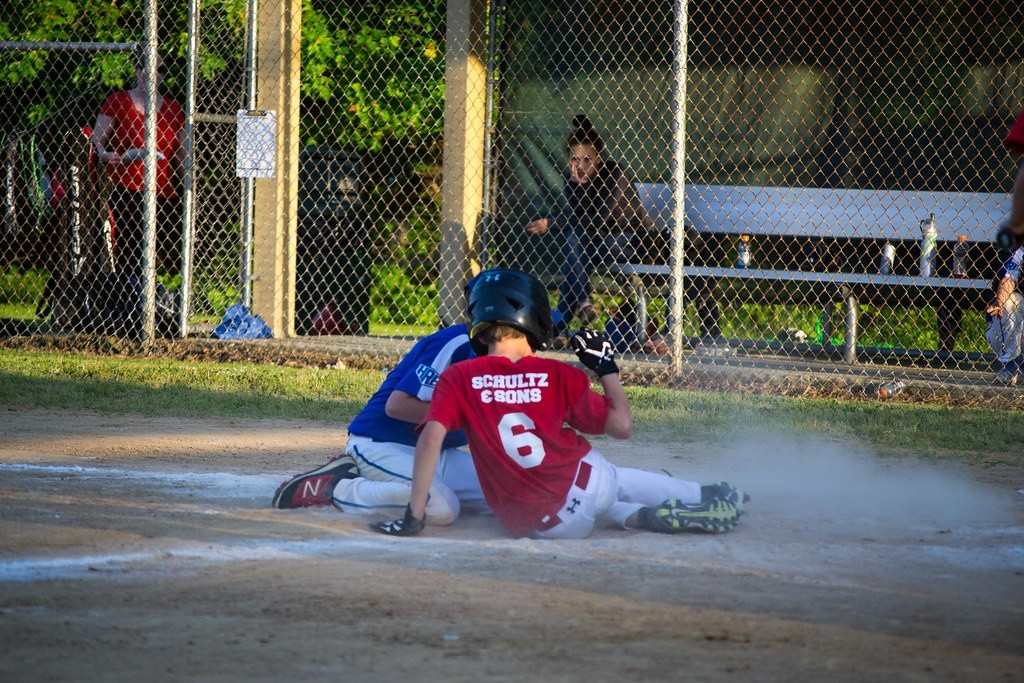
xmin=467 ymin=269 xmax=550 ymax=357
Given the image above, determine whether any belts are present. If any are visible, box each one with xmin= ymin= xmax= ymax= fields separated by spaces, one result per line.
xmin=537 ymin=461 xmax=592 ymax=533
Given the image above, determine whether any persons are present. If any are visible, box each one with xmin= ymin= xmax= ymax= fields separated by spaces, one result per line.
xmin=986 ymin=105 xmax=1024 ymax=386
xmin=368 ymin=271 xmax=751 ymax=540
xmin=527 ymin=114 xmax=669 ymax=350
xmin=91 ymin=49 xmax=194 ymax=340
xmin=271 ymin=271 xmax=495 ymax=527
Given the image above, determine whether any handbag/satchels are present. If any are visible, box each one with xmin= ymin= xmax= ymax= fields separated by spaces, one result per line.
xmin=210 ymin=304 xmax=273 ymax=341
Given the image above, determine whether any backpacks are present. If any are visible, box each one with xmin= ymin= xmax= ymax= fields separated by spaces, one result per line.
xmin=36 ymin=265 xmax=179 ymax=341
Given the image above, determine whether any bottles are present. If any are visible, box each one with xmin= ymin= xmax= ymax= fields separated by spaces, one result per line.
xmin=954 ymin=235 xmax=970 ymax=278
xmin=696 ymin=344 xmax=738 ymax=358
xmin=879 ymin=380 xmax=906 ymax=396
xmin=918 ymin=213 xmax=938 ymax=277
xmin=738 ymin=234 xmax=752 ymax=269
xmin=881 ymin=245 xmax=896 ymax=273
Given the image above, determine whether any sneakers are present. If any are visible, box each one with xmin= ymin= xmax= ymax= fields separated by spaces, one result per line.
xmin=271 ymin=454 xmax=361 ymax=509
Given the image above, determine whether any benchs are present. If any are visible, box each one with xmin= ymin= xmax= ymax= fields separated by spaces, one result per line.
xmin=604 ymin=183 xmax=1014 ymax=366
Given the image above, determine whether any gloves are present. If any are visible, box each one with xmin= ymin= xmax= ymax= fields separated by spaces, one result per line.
xmin=368 ymin=501 xmax=426 ymax=536
xmin=570 ymin=326 xmax=620 ymax=378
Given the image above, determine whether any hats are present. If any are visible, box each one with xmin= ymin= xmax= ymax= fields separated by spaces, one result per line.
xmin=463 ymin=266 xmax=502 ymax=312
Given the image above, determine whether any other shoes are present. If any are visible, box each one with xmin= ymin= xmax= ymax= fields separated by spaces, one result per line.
xmin=635 ymin=330 xmax=669 ymax=354
xmin=696 ymin=335 xmax=730 ymax=353
xmin=708 ymin=480 xmax=751 ymax=507
xmin=646 ymin=496 xmax=741 ymax=533
xmin=554 ymin=334 xmax=570 ymax=350
xmin=991 ymin=368 xmax=1018 ymax=385
xmin=577 ymin=304 xmax=601 ymax=326
